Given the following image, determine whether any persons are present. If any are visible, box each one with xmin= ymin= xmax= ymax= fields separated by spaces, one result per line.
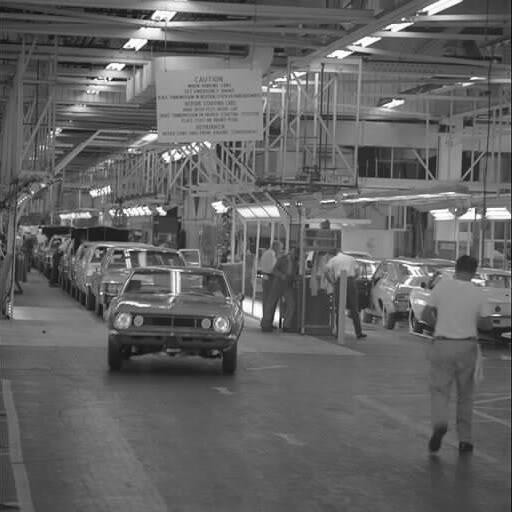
xmin=48 ymin=237 xmax=70 ymax=287
xmin=325 ymin=248 xmax=367 ymax=338
xmin=421 ymin=255 xmax=494 ymax=453
xmin=201 ymin=275 xmax=226 ymax=297
xmin=260 ymin=240 xmax=281 ymax=285
xmin=262 ymin=248 xmax=300 ymax=333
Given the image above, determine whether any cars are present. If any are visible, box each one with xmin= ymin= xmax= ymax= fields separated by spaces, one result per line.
xmin=356 ymin=259 xmax=454 ymax=330
xmin=91 ymin=246 xmax=186 ymax=319
xmin=105 ymin=265 xmax=244 ymax=375
xmin=31 ymin=235 xmax=154 ymax=309
xmin=408 ymin=267 xmax=511 ymax=339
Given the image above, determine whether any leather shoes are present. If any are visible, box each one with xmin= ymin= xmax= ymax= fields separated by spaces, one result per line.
xmin=428 ymin=423 xmax=448 ymax=451
xmin=357 ymin=333 xmax=367 ymax=339
xmin=458 ymin=441 xmax=474 ymax=453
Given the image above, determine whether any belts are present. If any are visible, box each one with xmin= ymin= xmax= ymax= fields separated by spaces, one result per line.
xmin=434 ymin=335 xmax=478 ymax=340
